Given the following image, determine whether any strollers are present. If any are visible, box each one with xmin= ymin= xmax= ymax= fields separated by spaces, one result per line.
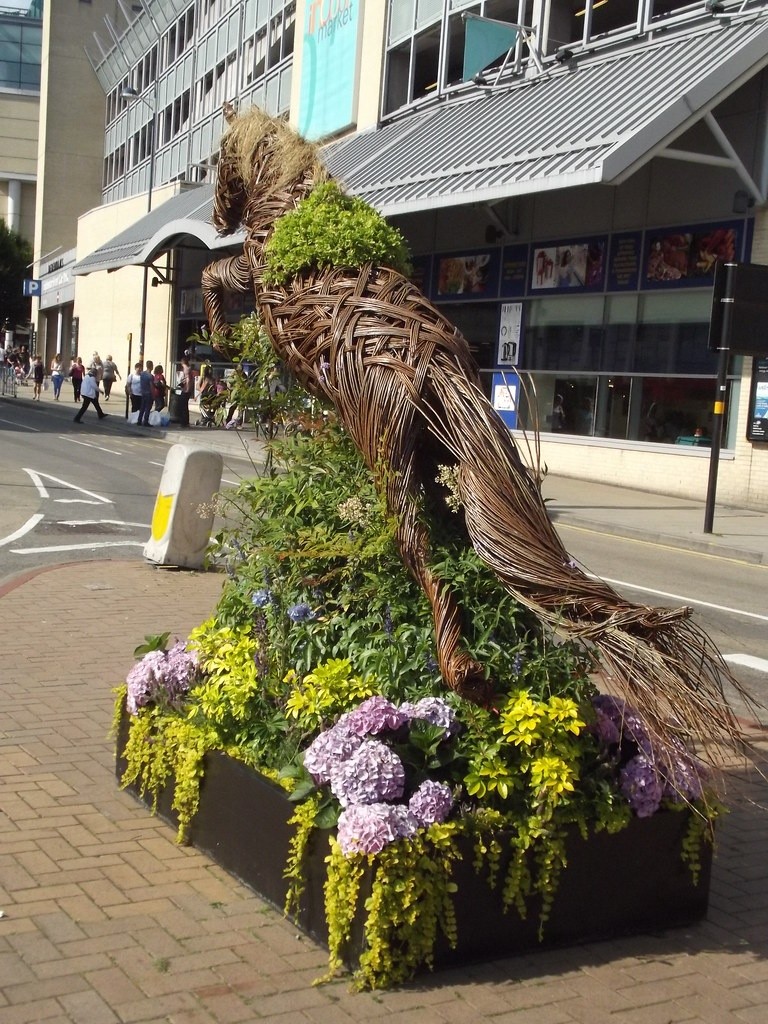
xmin=10 ymin=362 xmax=29 ymax=387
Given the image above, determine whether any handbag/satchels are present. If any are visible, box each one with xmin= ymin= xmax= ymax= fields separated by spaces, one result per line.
xmin=148 ymin=411 xmax=161 ymax=426
xmin=68 ymin=369 xmax=74 ymax=377
xmin=158 ymin=407 xmax=170 ymax=426
xmin=127 ymin=410 xmax=145 ymax=424
xmin=112 ymin=375 xmax=117 ymax=382
xmin=125 ymin=374 xmax=133 ymax=395
xmin=43 ymin=378 xmax=49 ymax=391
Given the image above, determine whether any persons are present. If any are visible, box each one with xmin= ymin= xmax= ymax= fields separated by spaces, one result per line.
xmin=86 ymin=354 xmax=104 ymax=399
xmin=176 ymin=357 xmax=193 ymax=429
xmin=73 ymin=368 xmax=109 ymax=425
xmin=153 ymin=365 xmax=171 ymax=413
xmin=102 ymin=354 xmax=122 ymax=401
xmin=50 ymin=353 xmax=66 ymax=402
xmin=641 ymin=394 xmax=663 ymax=442
xmin=126 ymin=363 xmax=144 ymax=413
xmin=558 ymin=248 xmax=574 ymax=287
xmin=0 ymin=343 xmax=31 ymax=388
xmin=89 ymin=350 xmax=103 ymax=365
xmin=194 ymin=363 xmax=309 ymax=435
xmin=25 ymin=354 xmax=48 ymax=402
xmin=542 ymin=393 xmax=565 ymax=433
xmin=136 ymin=361 xmax=155 ymax=427
xmin=68 ymin=356 xmax=86 ymax=403
xmin=577 ymin=397 xmax=596 ymax=436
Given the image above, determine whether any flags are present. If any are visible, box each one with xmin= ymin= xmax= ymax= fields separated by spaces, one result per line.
xmin=462 ymin=18 xmax=518 ymax=83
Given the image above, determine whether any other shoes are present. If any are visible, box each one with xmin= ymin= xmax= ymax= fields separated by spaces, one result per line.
xmin=33 ymin=394 xmax=40 ymax=401
xmin=225 ymin=426 xmax=230 ymax=430
xmin=237 ymin=426 xmax=244 ymax=430
xmin=73 ymin=419 xmax=84 ymax=424
xmin=105 ymin=396 xmax=108 ymax=400
xmin=53 ymin=396 xmax=58 ymax=402
xmin=207 ymin=418 xmax=213 ymax=428
xmin=75 ymin=398 xmax=81 ymax=402
xmin=98 ymin=414 xmax=108 ymax=419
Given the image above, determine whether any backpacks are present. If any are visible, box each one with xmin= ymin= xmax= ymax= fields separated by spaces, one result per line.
xmin=33 ymin=362 xmax=44 ymax=379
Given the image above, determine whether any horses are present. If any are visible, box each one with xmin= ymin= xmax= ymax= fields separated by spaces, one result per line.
xmin=200 ymin=100 xmax=768 ymax=826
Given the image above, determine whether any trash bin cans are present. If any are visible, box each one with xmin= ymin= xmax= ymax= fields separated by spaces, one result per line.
xmin=168 ymin=386 xmax=189 ymax=423
